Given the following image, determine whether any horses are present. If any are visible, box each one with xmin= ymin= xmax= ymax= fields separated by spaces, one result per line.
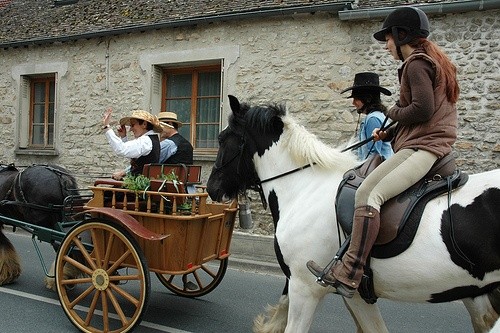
xmin=207 ymin=94 xmax=499 ymax=332
xmin=0 ymin=163 xmax=86 ymax=290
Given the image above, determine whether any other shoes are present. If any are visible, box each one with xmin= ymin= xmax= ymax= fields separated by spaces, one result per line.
xmin=307 ymin=260 xmax=356 ymax=298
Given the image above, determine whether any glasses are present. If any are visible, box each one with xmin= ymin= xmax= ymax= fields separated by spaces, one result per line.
xmin=130 ymin=122 xmax=141 ymax=127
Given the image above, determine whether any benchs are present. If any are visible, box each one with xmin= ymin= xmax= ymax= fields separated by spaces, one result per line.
xmin=92 ymin=163 xmax=203 ymax=211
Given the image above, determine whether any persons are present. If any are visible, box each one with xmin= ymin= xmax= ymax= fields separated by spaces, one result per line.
xmin=340 ymin=72 xmax=394 ymax=159
xmin=306 ymin=5 xmax=460 ymax=298
xmin=102 ymin=108 xmax=163 ymax=197
xmin=112 ymin=111 xmax=195 ymax=194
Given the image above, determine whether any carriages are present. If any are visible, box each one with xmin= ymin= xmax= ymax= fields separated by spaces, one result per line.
xmin=0 ymin=161 xmax=238 ymax=333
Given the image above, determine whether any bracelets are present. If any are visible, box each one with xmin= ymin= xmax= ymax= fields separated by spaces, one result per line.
xmin=104 ymin=125 xmax=110 ymax=130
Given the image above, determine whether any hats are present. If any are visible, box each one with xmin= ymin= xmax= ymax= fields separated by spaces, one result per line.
xmin=341 ymin=72 xmax=391 ymax=96
xmin=347 ymin=88 xmax=380 ymax=98
xmin=120 ymin=110 xmax=164 ymax=129
xmin=158 ymin=112 xmax=183 ymax=126
xmin=373 ymin=7 xmax=430 ymax=46
